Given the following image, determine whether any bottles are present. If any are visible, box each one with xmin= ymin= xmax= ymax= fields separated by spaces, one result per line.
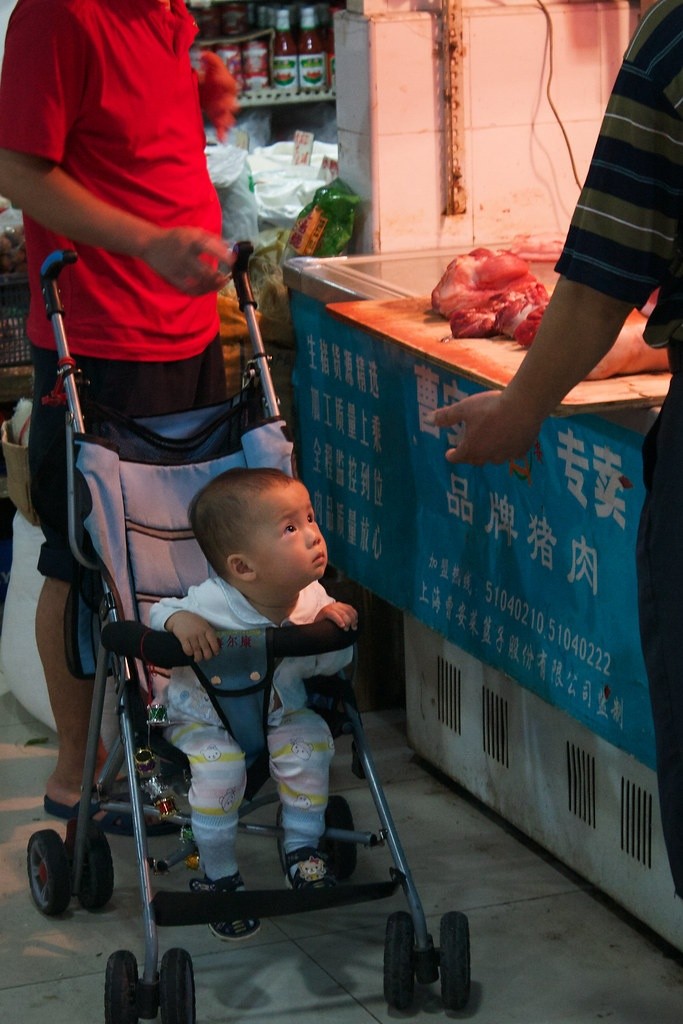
xmin=249 ymin=0 xmax=343 ymax=89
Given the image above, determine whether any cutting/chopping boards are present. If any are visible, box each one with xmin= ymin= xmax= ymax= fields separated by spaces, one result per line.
xmin=325 ymin=299 xmax=675 ymax=418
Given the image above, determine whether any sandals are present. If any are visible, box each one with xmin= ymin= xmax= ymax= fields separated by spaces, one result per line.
xmin=285 ymin=846 xmax=337 ymax=891
xmin=189 ymin=871 xmax=261 ymax=943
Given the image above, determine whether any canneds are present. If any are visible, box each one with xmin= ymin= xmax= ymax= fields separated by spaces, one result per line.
xmin=188 ymin=38 xmax=269 ymax=94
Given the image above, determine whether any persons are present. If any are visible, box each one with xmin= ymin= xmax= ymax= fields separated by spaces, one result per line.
xmin=0 ymin=0 xmax=243 ymax=834
xmin=426 ymin=0 xmax=683 ymax=896
xmin=142 ymin=467 xmax=358 ymax=940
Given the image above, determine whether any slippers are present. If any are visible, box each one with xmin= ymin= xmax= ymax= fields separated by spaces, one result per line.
xmin=43 ymin=781 xmax=188 ymax=837
xmin=110 ymin=755 xmax=183 ymax=789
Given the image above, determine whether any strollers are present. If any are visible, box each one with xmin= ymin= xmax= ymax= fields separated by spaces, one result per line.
xmin=24 ymin=231 xmax=475 ymax=1024
